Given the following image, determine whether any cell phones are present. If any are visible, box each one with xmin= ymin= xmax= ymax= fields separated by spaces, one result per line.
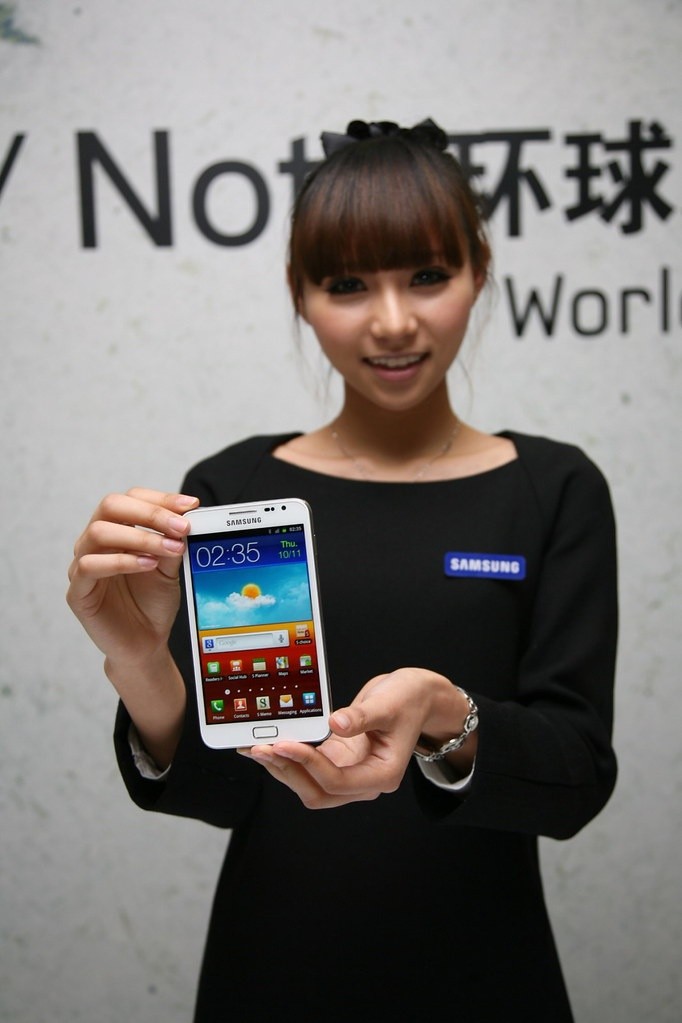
xmin=179 ymin=498 xmax=333 ymax=749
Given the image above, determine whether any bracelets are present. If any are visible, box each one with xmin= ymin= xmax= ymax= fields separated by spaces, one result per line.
xmin=411 ymin=685 xmax=478 ymax=762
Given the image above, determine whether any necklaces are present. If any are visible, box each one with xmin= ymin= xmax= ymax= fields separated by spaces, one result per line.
xmin=330 ymin=422 xmax=461 ymax=481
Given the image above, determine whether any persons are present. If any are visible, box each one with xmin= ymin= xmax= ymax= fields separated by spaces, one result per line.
xmin=66 ymin=118 xmax=618 ymax=1023
